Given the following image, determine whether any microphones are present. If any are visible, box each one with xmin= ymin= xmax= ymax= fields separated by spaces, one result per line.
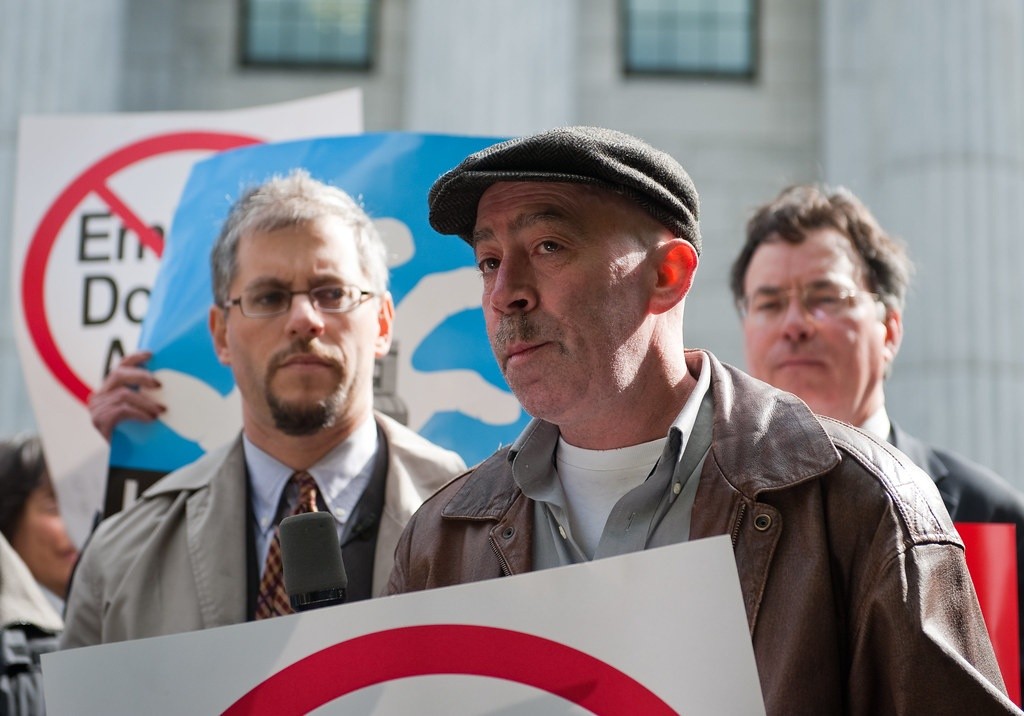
xmin=280 ymin=512 xmax=347 ymax=611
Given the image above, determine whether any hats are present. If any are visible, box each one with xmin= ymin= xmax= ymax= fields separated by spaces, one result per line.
xmin=428 ymin=124 xmax=704 ymax=257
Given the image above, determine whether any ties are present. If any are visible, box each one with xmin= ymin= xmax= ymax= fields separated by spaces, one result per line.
xmin=252 ymin=467 xmax=323 ymax=623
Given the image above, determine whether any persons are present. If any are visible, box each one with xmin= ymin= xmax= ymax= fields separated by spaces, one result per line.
xmin=59 ymin=171 xmax=470 ymax=650
xmin=0 ymin=352 xmax=168 ymax=716
xmin=379 ymin=126 xmax=1009 ymax=716
xmin=730 ymin=183 xmax=1024 ymax=716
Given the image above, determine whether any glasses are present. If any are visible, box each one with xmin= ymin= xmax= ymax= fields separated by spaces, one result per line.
xmin=220 ymin=282 xmax=378 ymax=316
xmin=734 ymin=279 xmax=880 ymax=324
